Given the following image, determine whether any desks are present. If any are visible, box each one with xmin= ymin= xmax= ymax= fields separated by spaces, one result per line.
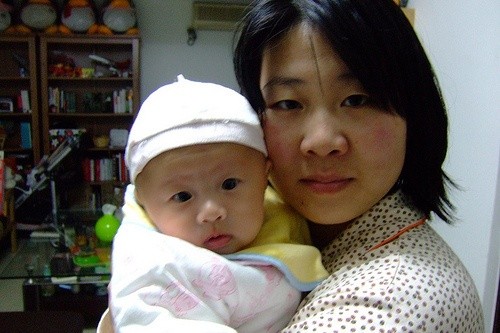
xmin=1 ymin=208 xmax=112 ymax=325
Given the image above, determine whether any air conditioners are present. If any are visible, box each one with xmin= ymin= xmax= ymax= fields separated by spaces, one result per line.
xmin=191 ymin=3 xmax=255 ymax=31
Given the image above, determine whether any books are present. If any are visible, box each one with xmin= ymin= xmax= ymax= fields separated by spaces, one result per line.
xmin=0 ymin=54 xmax=134 ymax=214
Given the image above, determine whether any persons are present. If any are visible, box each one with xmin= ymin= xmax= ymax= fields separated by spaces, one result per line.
xmin=232 ymin=0 xmax=486 ymax=333
xmin=97 ymin=74 xmax=329 ymax=333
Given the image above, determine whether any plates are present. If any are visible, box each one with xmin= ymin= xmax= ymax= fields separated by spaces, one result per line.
xmin=103 ymin=8 xmax=136 ymax=31
xmin=22 ymin=4 xmax=56 ymax=29
xmin=62 ymin=6 xmax=95 ymax=32
xmin=0 ymin=10 xmax=10 ymax=31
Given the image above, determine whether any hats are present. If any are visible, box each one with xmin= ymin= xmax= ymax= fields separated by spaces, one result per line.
xmin=123 ymin=73 xmax=268 ymax=185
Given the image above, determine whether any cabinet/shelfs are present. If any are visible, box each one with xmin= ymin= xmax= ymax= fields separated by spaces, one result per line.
xmin=0 ymin=32 xmax=142 ymax=253
